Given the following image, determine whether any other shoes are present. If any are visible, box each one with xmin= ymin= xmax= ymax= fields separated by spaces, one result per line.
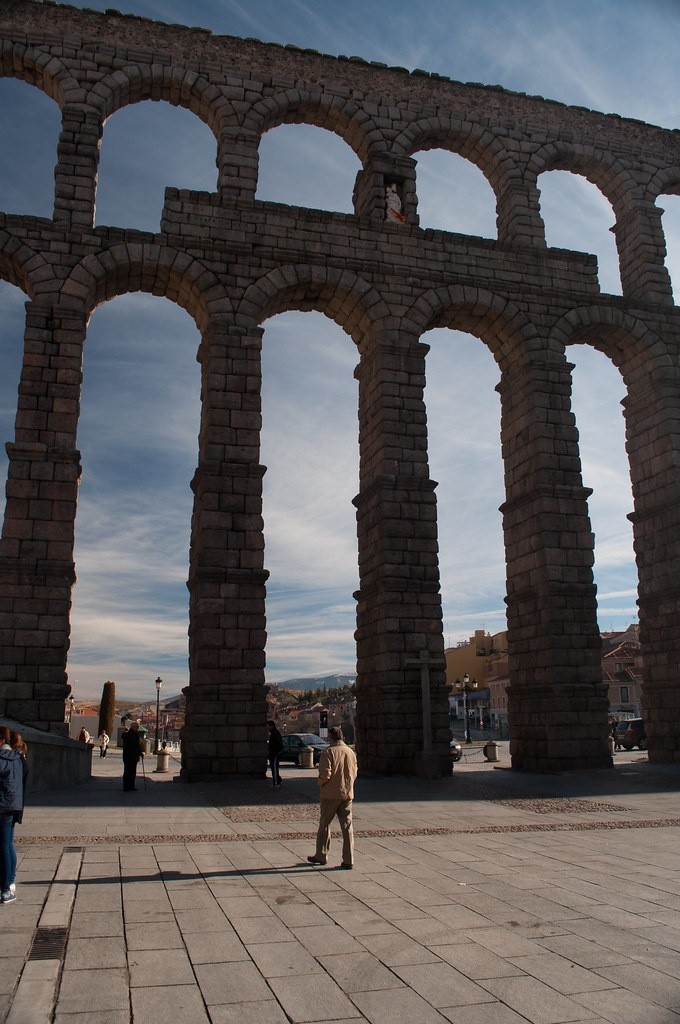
xmin=308 ymin=853 xmax=325 ymax=866
xmin=130 ymin=788 xmax=137 ymax=791
xmin=1 ymin=889 xmax=16 ymax=904
xmin=341 ymin=859 xmax=353 ymax=870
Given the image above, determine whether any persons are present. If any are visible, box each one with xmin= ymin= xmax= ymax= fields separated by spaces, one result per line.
xmin=99 ymin=730 xmax=109 ymax=759
xmin=162 ymin=741 xmax=166 ymax=749
xmin=386 ymin=184 xmax=401 ymax=222
xmin=76 ymin=727 xmax=90 ymax=743
xmin=0 ymin=725 xmax=28 ymax=903
xmin=307 ymin=726 xmax=357 ymax=868
xmin=266 ymin=720 xmax=283 ymax=790
xmin=120 ymin=723 xmax=144 ymax=792
xmin=609 ymin=718 xmax=621 ymax=752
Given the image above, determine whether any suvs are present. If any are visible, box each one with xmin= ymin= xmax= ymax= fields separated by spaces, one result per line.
xmin=614 ymin=717 xmax=648 ymax=751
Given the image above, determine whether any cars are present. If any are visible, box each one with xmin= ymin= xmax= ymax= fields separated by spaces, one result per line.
xmin=450 ymin=741 xmax=462 ymax=762
xmin=267 ymin=732 xmax=330 ymax=765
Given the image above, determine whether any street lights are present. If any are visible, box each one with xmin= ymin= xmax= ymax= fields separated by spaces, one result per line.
xmin=153 ymin=675 xmax=164 ymax=754
xmin=454 ymin=673 xmax=478 ymax=744
xmin=69 ymin=694 xmax=74 ymax=724
